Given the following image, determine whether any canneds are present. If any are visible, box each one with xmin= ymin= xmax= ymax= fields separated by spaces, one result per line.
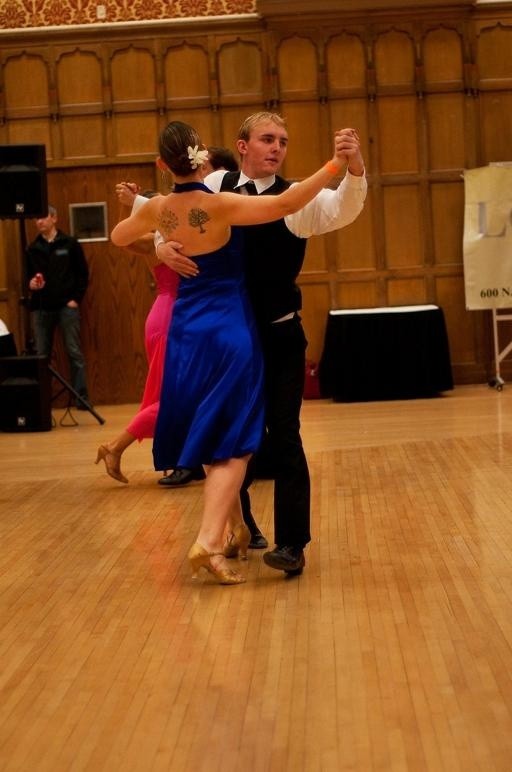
xmin=35 ymin=273 xmax=44 ymax=289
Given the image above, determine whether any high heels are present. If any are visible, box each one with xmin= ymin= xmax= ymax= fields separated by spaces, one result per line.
xmin=95 ymin=444 xmax=129 ymax=483
xmin=221 ymin=522 xmax=252 ymax=561
xmin=187 ymin=541 xmax=247 ymax=585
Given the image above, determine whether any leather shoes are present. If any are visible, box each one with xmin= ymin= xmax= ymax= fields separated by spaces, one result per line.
xmin=158 ymin=465 xmax=207 ymax=485
xmin=263 ymin=545 xmax=305 ymax=572
xmin=248 ymin=534 xmax=268 ymax=549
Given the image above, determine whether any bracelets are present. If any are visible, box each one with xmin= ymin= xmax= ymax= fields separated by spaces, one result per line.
xmin=325 ymin=160 xmax=338 ymax=176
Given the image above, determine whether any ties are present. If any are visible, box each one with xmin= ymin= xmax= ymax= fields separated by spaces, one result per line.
xmin=242 ymin=183 xmax=257 ymax=196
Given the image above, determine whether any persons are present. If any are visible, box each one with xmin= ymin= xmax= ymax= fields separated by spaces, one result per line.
xmin=152 ymin=111 xmax=368 ymax=573
xmin=109 ymin=122 xmax=356 ymax=585
xmin=20 ymin=204 xmax=91 ymax=410
xmin=115 ymin=149 xmax=237 ymax=485
xmin=94 ymin=182 xmax=177 ymax=484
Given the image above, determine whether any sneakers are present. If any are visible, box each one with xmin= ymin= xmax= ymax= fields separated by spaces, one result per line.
xmin=73 ymin=393 xmax=95 ymax=410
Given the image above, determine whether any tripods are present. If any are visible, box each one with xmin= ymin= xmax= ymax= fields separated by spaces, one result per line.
xmin=7 ymin=231 xmax=109 ymax=427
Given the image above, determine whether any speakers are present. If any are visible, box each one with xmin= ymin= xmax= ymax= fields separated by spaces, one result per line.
xmin=0 ymin=354 xmax=53 ymax=434
xmin=0 ymin=142 xmax=49 ymax=220
